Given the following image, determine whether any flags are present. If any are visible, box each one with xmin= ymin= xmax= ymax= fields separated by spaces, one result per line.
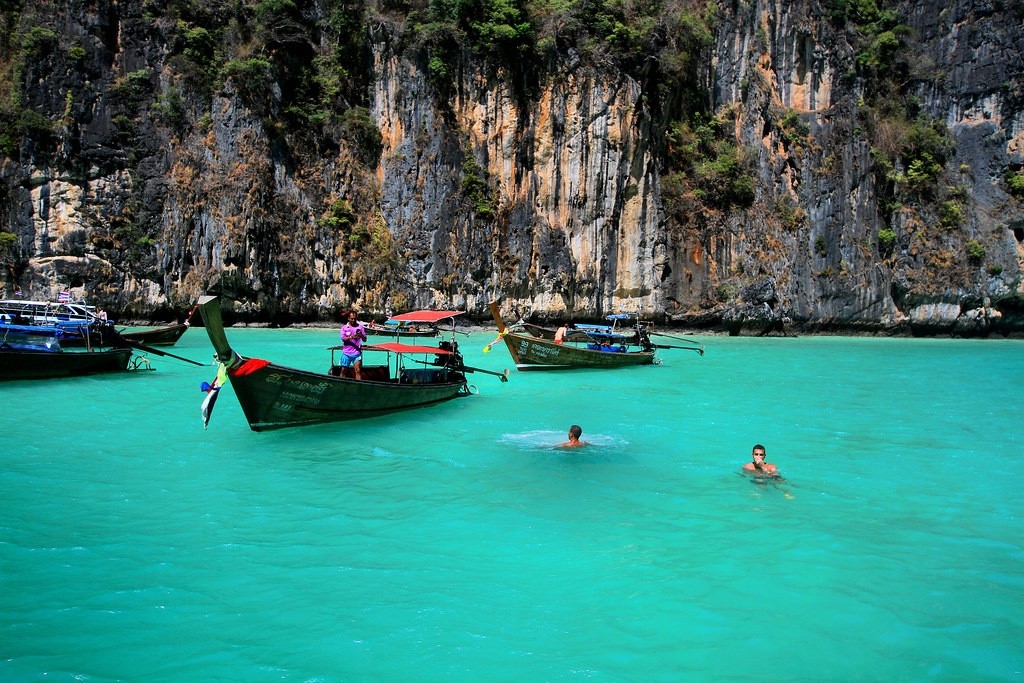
xmin=58 ymin=291 xmax=69 ymax=303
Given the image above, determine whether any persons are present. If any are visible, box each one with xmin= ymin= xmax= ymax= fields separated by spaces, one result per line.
xmin=535 ymin=332 xmax=545 ymax=340
xmin=97 ymin=307 xmax=107 ymax=325
xmin=744 ymin=444 xmax=777 ymax=476
xmin=340 ymin=311 xmax=367 ymax=380
xmin=556 ymin=425 xmax=590 ymax=447
xmin=601 ymin=337 xmax=614 ymax=347
xmin=0 ymin=315 xmax=6 ymax=324
xmin=554 ymin=324 xmax=569 ymax=345
xmin=367 ymin=320 xmax=414 ymax=332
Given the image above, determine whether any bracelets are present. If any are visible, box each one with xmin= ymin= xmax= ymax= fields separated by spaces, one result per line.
xmin=350 ymin=336 xmax=352 ymax=339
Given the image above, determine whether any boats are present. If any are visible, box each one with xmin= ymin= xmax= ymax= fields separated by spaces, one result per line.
xmin=356 ymin=320 xmax=439 ymax=337
xmin=197 ymin=295 xmax=469 ymax=432
xmin=488 ymin=300 xmax=656 ymax=371
xmin=518 ymin=320 xmax=642 ymax=345
xmin=0 ymin=299 xmax=195 ymax=347
xmin=0 ymin=341 xmax=132 ymax=379
